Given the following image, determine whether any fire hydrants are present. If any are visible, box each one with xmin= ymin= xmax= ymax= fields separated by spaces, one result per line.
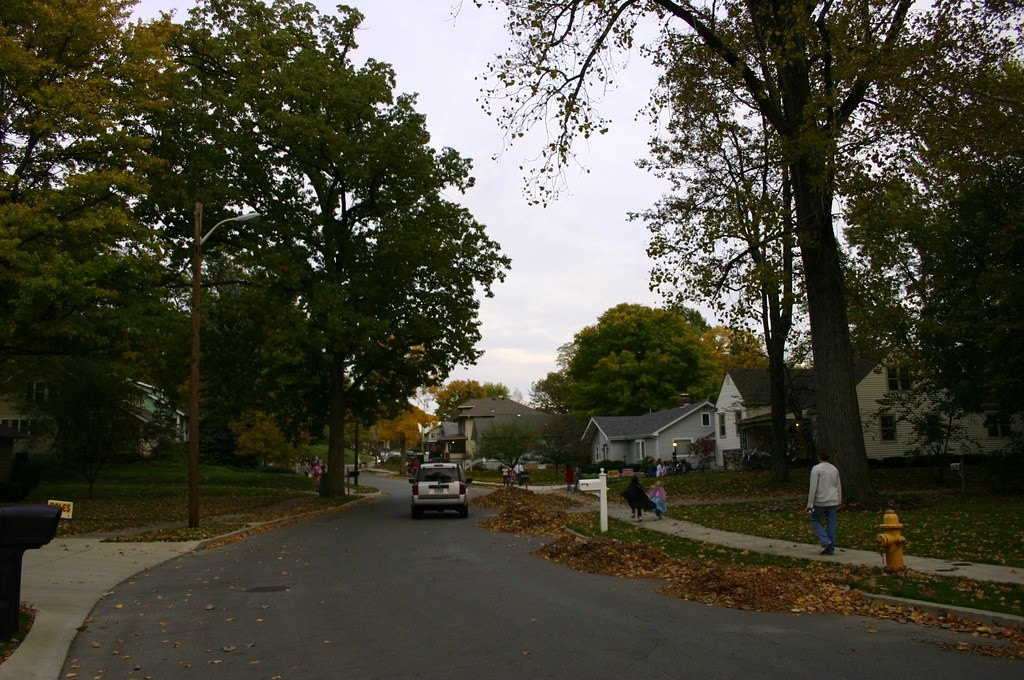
xmin=875 ymin=499 xmax=907 ymax=576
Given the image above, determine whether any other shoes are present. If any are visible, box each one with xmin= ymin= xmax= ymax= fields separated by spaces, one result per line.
xmin=631 ymin=514 xmax=636 ymax=518
xmin=819 ymin=541 xmax=832 ymax=554
xmin=638 ymin=517 xmax=642 ymax=522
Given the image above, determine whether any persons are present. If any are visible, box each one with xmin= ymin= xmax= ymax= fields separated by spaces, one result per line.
xmin=644 ymin=459 xmax=669 ymax=477
xmin=407 ymin=458 xmax=419 ymax=476
xmin=574 ymin=464 xmax=582 ymax=493
xmin=645 ymin=480 xmax=666 ymax=519
xmin=304 ymin=456 xmax=325 ymax=481
xmin=501 ymin=463 xmax=524 ymax=486
xmin=621 ymin=476 xmax=655 ymax=521
xmin=806 ymin=449 xmax=842 ymax=555
xmin=565 ymin=464 xmax=575 ymax=493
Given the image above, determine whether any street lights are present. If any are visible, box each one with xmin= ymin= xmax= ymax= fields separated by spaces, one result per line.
xmin=187 ymin=202 xmax=262 ymax=528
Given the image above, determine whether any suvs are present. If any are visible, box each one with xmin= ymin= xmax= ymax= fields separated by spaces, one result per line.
xmin=408 ymin=463 xmax=473 ymax=520
